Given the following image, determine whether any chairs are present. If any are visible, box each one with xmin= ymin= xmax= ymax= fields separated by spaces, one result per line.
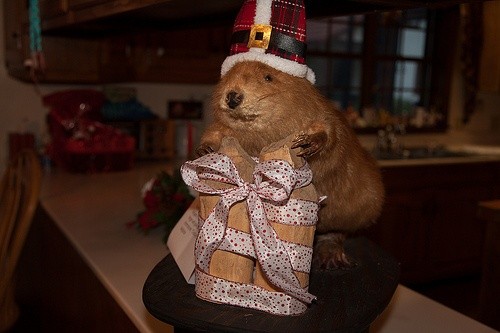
xmin=0 ymin=149 xmax=48 ymax=333
